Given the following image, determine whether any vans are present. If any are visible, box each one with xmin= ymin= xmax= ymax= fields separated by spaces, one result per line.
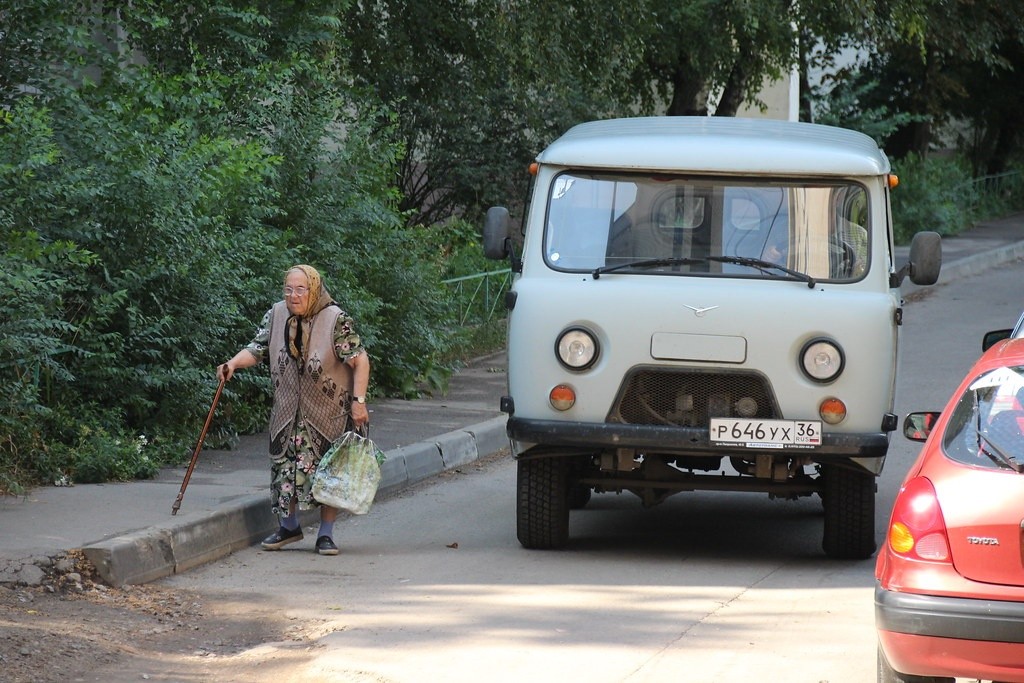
xmin=484 ymin=116 xmax=943 ymax=556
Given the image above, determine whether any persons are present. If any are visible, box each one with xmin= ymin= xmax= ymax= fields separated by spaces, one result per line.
xmin=216 ymin=264 xmax=370 ymax=554
xmin=759 ymin=188 xmax=868 ymax=279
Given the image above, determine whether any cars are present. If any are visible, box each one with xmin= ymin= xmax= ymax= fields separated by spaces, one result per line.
xmin=873 ymin=338 xmax=1023 ymax=683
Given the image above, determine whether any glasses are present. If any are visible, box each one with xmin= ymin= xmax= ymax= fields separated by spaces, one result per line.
xmin=283 ymin=287 xmax=309 ymax=297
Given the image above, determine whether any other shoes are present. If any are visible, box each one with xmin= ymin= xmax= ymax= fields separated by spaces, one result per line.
xmin=314 ymin=535 xmax=339 ymax=555
xmin=260 ymin=526 xmax=304 ymax=549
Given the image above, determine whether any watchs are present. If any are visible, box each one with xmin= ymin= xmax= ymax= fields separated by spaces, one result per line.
xmin=353 ymin=396 xmax=367 ymax=403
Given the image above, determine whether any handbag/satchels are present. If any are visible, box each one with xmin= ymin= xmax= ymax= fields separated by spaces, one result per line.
xmin=312 ymin=419 xmax=386 ymax=515
